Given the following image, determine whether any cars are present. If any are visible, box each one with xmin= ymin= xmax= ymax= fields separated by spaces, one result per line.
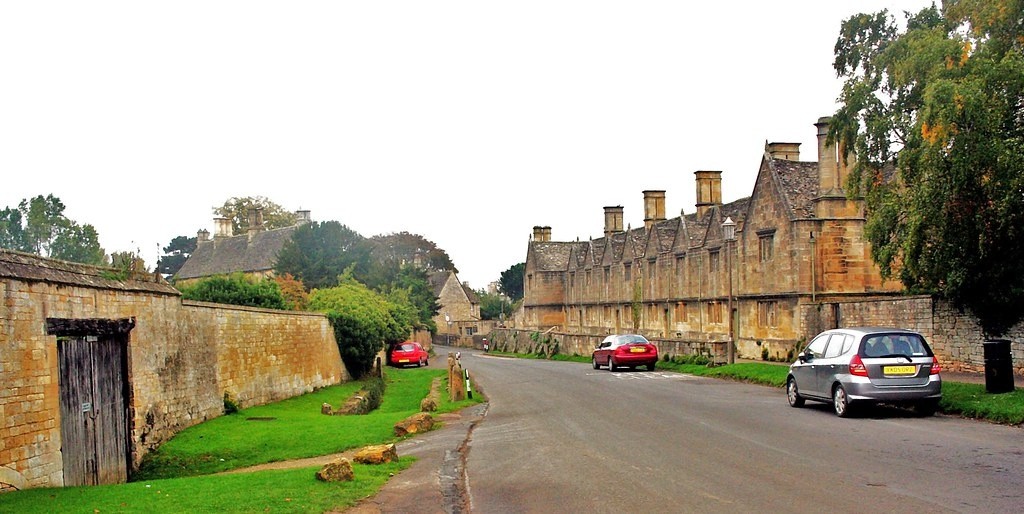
xmin=391 ymin=342 xmax=429 ymax=368
xmin=785 ymin=326 xmax=943 ymax=418
xmin=592 ymin=334 xmax=658 ymax=372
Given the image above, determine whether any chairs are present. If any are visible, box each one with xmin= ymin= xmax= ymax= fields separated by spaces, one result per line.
xmin=871 ymin=342 xmax=888 ymax=355
xmin=894 ymin=342 xmax=910 ymax=353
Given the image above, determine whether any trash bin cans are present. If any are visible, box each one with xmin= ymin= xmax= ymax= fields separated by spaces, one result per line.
xmin=983 ymin=339 xmax=1014 ymax=392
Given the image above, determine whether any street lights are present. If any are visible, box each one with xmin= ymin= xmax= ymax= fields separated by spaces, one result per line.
xmin=720 ymin=216 xmax=739 ymax=364
